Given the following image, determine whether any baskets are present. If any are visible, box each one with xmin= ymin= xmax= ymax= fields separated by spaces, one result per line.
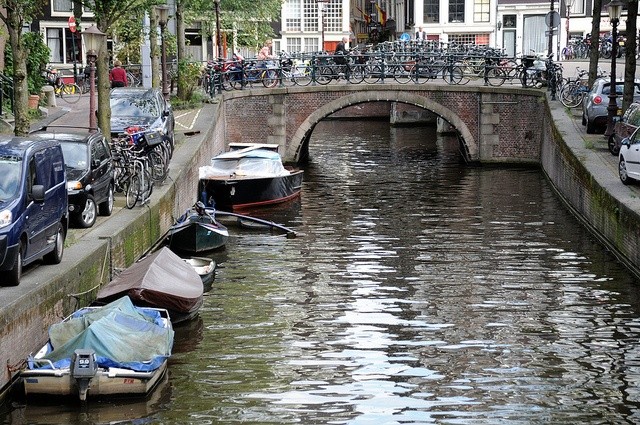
xmin=137 ymin=131 xmax=163 ymax=151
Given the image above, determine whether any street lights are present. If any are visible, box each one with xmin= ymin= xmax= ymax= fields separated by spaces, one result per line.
xmin=79 ymin=23 xmax=106 ymax=131
xmin=213 ymin=0 xmax=221 ymax=59
xmin=604 ymin=0 xmax=625 ymax=145
xmin=156 ymin=6 xmax=169 ymax=99
xmin=321 ymin=8 xmax=327 ymax=50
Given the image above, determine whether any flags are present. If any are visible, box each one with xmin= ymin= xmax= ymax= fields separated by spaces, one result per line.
xmin=375 ymin=3 xmax=386 ymax=27
xmin=357 ymin=7 xmax=371 ymax=24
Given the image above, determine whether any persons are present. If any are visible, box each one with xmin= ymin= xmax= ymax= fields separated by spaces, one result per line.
xmin=233 ymin=46 xmax=245 ymax=64
xmin=41 ymin=63 xmax=57 ymax=96
xmin=109 ymin=59 xmax=128 ymax=87
xmin=334 ymin=37 xmax=350 ymax=82
xmin=257 ymin=39 xmax=276 ymax=63
xmin=415 ymin=25 xmax=427 ymax=40
xmin=91 ymin=55 xmax=99 ymax=85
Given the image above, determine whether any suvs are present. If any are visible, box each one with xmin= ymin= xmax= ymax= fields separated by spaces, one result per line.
xmin=0 ymin=136 xmax=68 ymax=284
xmin=29 ymin=124 xmax=114 ymax=226
xmin=96 ymin=86 xmax=174 ymax=143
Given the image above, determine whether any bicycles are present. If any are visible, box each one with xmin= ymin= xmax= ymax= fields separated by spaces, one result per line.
xmin=572 ymin=33 xmax=640 ymax=59
xmin=362 ymin=56 xmax=412 ymax=83
xmin=109 ymin=126 xmax=174 ymax=208
xmin=203 ymin=53 xmax=313 ymax=92
xmin=452 ymin=56 xmax=506 ymax=85
xmin=313 ymin=51 xmax=365 ymax=83
xmin=410 ymin=57 xmax=463 ymax=83
xmin=44 ymin=68 xmax=81 ymax=103
xmin=557 ymin=65 xmax=589 ymax=107
xmin=501 ymin=53 xmax=563 ymax=90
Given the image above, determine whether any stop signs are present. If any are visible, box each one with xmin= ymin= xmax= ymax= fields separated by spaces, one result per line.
xmin=68 ymin=16 xmax=77 ymax=32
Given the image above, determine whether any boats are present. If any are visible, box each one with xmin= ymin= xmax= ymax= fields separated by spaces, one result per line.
xmin=97 ymin=247 xmax=204 ymax=320
xmin=197 ymin=141 xmax=303 ymax=210
xmin=22 ymin=294 xmax=176 ymax=395
xmin=183 ymin=256 xmax=216 ymax=292
xmin=169 ymin=201 xmax=228 ymax=250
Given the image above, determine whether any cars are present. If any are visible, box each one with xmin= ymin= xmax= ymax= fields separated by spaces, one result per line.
xmin=618 ymin=125 xmax=640 ymax=183
xmin=582 ymin=77 xmax=640 ymax=133
xmin=608 ymin=101 xmax=640 ymax=154
